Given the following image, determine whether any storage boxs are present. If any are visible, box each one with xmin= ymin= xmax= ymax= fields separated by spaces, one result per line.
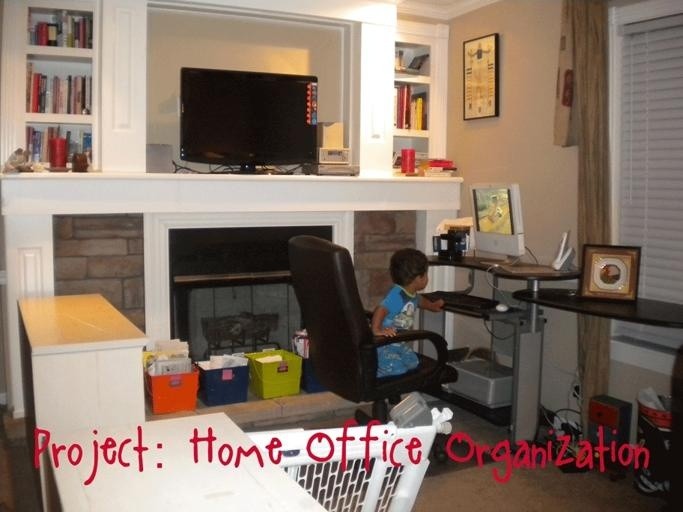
xmin=196 ymin=358 xmax=248 ymax=406
xmin=245 ymin=350 xmax=302 ymax=400
xmin=447 ymin=360 xmax=513 ymax=409
xmin=144 ymin=367 xmax=199 ymax=414
xmin=300 ymin=352 xmax=325 ymax=393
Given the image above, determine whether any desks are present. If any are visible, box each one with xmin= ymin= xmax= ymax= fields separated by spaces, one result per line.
xmin=412 ymin=254 xmax=581 ymax=427
xmin=509 ymin=287 xmax=682 ymax=476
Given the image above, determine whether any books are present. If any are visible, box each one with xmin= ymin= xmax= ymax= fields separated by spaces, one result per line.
xmin=142 ymin=328 xmax=310 ymax=377
xmin=415 ymin=158 xmax=458 ymax=178
xmin=25 ymin=8 xmax=92 ymax=169
xmin=394 ymin=48 xmax=429 ymax=177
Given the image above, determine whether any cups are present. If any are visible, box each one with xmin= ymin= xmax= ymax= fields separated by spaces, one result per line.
xmin=47 ymin=137 xmax=68 ymax=168
xmin=400 ymin=148 xmax=417 ymax=174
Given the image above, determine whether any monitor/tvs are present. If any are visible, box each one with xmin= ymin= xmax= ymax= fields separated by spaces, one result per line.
xmin=180 ymin=67 xmax=317 ymax=175
xmin=469 ymin=184 xmax=526 ymax=268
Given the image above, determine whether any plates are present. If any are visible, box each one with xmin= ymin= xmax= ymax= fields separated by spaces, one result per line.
xmin=43 ymin=166 xmax=72 ymax=172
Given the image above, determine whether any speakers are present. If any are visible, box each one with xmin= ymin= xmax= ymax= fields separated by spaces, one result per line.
xmin=317 ymin=122 xmax=344 ymax=148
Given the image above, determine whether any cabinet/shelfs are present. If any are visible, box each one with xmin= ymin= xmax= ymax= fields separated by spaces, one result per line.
xmin=393 ymin=18 xmax=449 ymax=172
xmin=0 ymin=1 xmax=104 ymax=170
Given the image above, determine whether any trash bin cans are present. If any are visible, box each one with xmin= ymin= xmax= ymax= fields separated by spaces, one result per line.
xmin=636 ymin=395 xmax=671 ymax=497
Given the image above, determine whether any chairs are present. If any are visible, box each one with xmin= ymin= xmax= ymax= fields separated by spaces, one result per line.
xmin=260 ymin=426 xmax=438 ymax=512
xmin=287 ymin=236 xmax=457 ymax=460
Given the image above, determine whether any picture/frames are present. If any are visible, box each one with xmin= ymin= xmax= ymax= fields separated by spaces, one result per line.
xmin=578 ymin=244 xmax=642 ymax=303
xmin=462 ymin=33 xmax=499 ymax=120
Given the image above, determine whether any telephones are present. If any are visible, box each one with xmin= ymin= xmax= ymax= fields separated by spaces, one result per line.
xmin=551 ymin=230 xmax=575 ymax=271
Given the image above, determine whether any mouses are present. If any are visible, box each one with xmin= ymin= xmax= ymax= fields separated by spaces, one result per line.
xmin=495 ymin=303 xmax=509 ymax=312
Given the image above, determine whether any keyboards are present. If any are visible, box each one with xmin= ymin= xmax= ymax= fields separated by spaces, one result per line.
xmin=429 ymin=291 xmax=499 ymax=310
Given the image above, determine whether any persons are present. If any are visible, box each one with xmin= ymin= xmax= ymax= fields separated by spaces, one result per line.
xmin=602 ymin=265 xmax=613 ymax=283
xmin=369 ymin=246 xmax=446 ymax=380
xmin=486 ymin=194 xmax=504 ymax=234
